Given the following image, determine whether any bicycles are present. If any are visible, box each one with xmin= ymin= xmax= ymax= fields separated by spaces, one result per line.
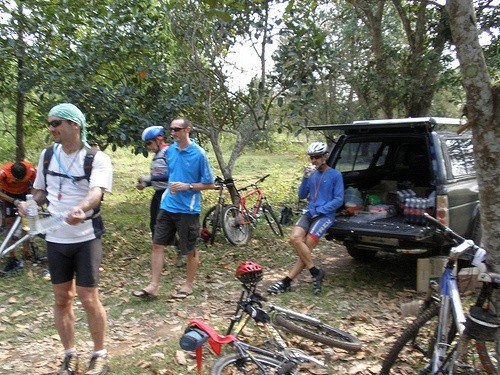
xmin=222 ymin=172 xmax=284 ymax=248
xmin=0 ymin=208 xmax=48 ymax=276
xmin=179 ymin=281 xmax=363 ymax=375
xmin=202 ymin=175 xmax=234 ymax=245
xmin=379 ymin=212 xmax=500 ymax=375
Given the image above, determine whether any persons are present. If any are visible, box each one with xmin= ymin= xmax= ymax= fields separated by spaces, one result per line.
xmin=18 ymin=103 xmax=113 ymax=375
xmin=132 ymin=117 xmax=215 ymax=303
xmin=0 ymin=160 xmax=37 ymax=277
xmin=266 ymin=142 xmax=344 ymax=295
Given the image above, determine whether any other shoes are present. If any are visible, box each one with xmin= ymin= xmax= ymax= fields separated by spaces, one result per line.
xmin=21 ymin=255 xmax=41 ymax=264
xmin=1 ymin=259 xmax=19 ymax=273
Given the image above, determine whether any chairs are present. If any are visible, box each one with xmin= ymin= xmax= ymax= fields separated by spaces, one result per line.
xmin=393 ymin=144 xmax=426 ymax=171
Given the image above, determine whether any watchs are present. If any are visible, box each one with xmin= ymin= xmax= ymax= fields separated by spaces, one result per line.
xmin=188 ymin=183 xmax=194 ymax=190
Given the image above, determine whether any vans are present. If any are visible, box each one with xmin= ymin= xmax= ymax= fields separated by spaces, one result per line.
xmin=306 ymin=116 xmax=480 ymax=261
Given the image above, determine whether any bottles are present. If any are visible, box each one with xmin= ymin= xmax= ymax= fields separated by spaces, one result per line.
xmin=26 ymin=194 xmax=40 ymax=234
xmin=404 ymin=197 xmax=433 ymax=225
xmin=35 ymin=208 xmax=78 ymax=234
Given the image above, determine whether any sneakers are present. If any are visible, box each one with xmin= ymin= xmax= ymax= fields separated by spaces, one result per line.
xmin=266 ymin=281 xmax=292 ymax=293
xmin=58 ymin=354 xmax=79 ymax=375
xmin=84 ymin=356 xmax=110 ymax=375
xmin=313 ymin=269 xmax=325 ymax=294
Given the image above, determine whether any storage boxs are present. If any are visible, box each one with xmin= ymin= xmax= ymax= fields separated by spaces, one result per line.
xmin=458 ymin=267 xmax=482 ymax=292
xmin=417 ymin=256 xmax=457 ymax=293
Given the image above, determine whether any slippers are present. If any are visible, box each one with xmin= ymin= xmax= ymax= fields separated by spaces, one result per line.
xmin=171 ymin=290 xmax=194 ymax=298
xmin=132 ymin=290 xmax=152 ymax=296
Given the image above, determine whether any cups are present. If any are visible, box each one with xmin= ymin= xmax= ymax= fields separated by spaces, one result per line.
xmin=169 ymin=182 xmax=176 ymax=195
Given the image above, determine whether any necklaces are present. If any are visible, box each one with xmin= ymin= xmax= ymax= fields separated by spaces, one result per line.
xmin=58 ymin=143 xmax=84 ymax=201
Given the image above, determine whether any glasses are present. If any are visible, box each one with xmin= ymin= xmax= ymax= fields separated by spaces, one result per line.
xmin=47 ymin=120 xmax=67 ymax=127
xmin=310 ymin=155 xmax=321 ymax=159
xmin=146 ymin=142 xmax=153 ymax=145
xmin=169 ymin=128 xmax=185 ymax=132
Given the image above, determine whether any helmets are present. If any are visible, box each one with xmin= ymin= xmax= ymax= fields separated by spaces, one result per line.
xmin=306 ymin=143 xmax=327 ymax=156
xmin=142 ymin=126 xmax=164 ymax=141
xmin=236 ymin=261 xmax=263 ymax=282
